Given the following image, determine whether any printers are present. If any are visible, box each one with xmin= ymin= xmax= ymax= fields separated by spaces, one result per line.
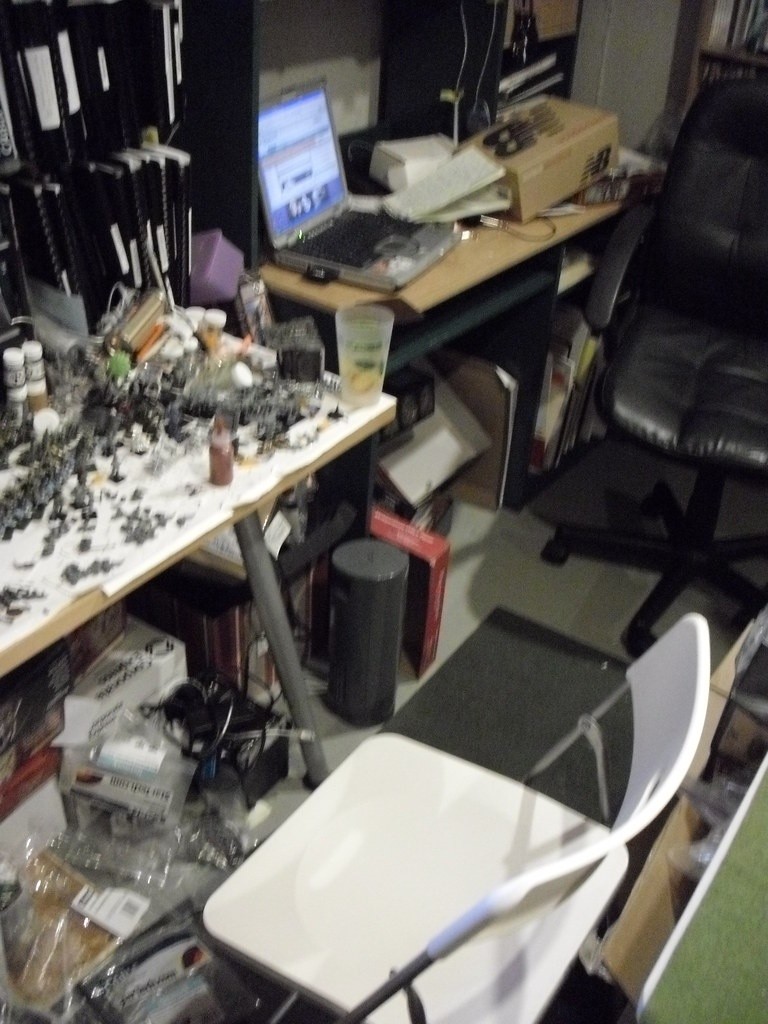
xmin=368 ymin=132 xmax=512 ymax=224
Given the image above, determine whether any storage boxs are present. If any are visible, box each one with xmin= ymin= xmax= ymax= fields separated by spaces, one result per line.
xmin=464 ymin=96 xmax=620 ymax=225
xmin=0 ymin=748 xmax=69 ymax=868
xmin=368 ymin=133 xmax=445 ymax=184
xmin=191 ymin=228 xmax=244 ymax=304
xmin=370 ymin=504 xmax=451 ymax=682
xmin=72 ymin=626 xmax=190 ymax=733
xmin=0 ymin=639 xmax=65 ymax=784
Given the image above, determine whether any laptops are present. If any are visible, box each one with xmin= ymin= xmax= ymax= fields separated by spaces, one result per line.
xmin=257 ymin=77 xmax=460 ymax=294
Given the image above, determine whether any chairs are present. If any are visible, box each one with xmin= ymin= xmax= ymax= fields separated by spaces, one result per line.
xmin=539 ymin=79 xmax=768 ymax=659
xmin=201 ymin=611 xmax=711 ymax=1024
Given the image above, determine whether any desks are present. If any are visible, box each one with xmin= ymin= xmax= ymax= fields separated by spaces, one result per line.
xmin=0 ymin=332 xmax=399 ymax=794
xmin=258 ymin=200 xmax=622 ymax=541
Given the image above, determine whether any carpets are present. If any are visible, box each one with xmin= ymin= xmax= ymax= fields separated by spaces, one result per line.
xmin=377 ymin=604 xmax=633 ymax=828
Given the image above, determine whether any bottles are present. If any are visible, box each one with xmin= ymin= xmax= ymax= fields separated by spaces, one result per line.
xmin=22 ymin=341 xmax=44 ymax=381
xmin=210 ymin=421 xmax=233 ymax=484
xmin=201 ymin=309 xmax=227 ymax=355
xmin=7 ymin=386 xmax=29 ymax=418
xmin=3 ymin=348 xmax=25 ymax=388
xmin=26 ymin=379 xmax=49 ymax=412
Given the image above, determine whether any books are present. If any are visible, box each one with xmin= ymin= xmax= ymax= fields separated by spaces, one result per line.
xmin=522 ymin=302 xmax=617 ymax=479
xmin=1 ymin=0 xmax=195 ymax=393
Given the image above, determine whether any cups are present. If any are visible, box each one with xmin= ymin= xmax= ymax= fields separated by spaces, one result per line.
xmin=336 ymin=306 xmax=395 ymax=408
xmin=111 ymin=288 xmax=169 ymax=355
